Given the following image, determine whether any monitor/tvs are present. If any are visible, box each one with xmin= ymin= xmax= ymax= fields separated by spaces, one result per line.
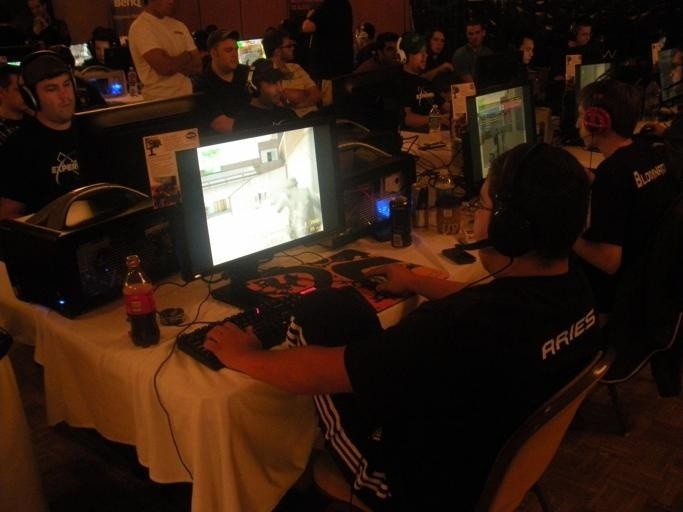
xmin=330 ymin=65 xmax=403 ymax=127
xmin=656 ymin=44 xmax=682 ymax=103
xmin=573 ymin=60 xmax=617 ymax=95
xmin=234 ymin=36 xmax=271 ymax=73
xmin=170 ymin=112 xmax=347 ymax=311
xmin=474 ymin=50 xmax=523 ymax=77
xmin=70 ymin=41 xmax=96 ymax=67
xmin=72 ymin=90 xmax=212 ymax=219
xmin=465 ymin=79 xmax=543 ymax=186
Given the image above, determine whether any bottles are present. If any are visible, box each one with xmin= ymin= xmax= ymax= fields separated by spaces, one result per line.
xmin=435 ymin=167 xmax=461 ymax=234
xmin=124 ymin=255 xmax=160 ymax=347
xmin=127 ymin=65 xmax=138 ymax=98
xmin=427 ymin=103 xmax=442 ymax=145
xmin=390 ymin=196 xmax=411 ymax=248
xmin=411 ymin=181 xmax=429 ymax=228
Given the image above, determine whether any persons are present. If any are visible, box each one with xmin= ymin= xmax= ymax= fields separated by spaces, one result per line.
xmin=128 ymin=0 xmax=203 ymax=98
xmin=354 ymin=31 xmax=403 ymax=74
xmin=422 ymin=29 xmax=452 ymax=83
xmin=492 ymin=31 xmax=536 ymax=71
xmin=191 ymin=28 xmax=255 ymax=133
xmin=354 ymin=23 xmax=376 ymax=69
xmin=303 ymin=1 xmax=354 ymax=126
xmin=84 ymin=28 xmax=110 ymax=69
xmin=452 ymin=22 xmax=491 ymax=81
xmin=572 ymin=80 xmax=683 ymax=323
xmin=1 ymin=63 xmax=31 ymax=124
xmin=1 ymin=50 xmax=127 ymax=212
xmin=204 ymin=144 xmax=608 ymax=506
xmin=384 ymin=36 xmax=451 ymax=130
xmin=28 ymin=5 xmax=72 ymax=51
xmin=252 ymin=34 xmax=322 ymax=118
xmin=555 ymin=21 xmax=592 ymax=61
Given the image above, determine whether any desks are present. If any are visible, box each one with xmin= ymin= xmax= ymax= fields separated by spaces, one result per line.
xmin=0 ymin=72 xmax=204 ymax=132
xmin=0 ymin=97 xmax=680 ymax=498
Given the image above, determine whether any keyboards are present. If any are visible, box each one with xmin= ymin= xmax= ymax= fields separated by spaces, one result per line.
xmin=177 ymin=290 xmax=311 ymax=372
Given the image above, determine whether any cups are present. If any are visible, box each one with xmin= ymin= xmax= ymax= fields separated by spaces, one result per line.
xmin=459 ymin=205 xmax=478 ymax=245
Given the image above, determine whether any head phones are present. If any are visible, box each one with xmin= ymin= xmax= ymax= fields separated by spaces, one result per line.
xmin=394 ymin=32 xmax=409 ymax=65
xmin=582 ymin=78 xmax=616 ymax=135
xmin=17 ymin=50 xmax=81 ymax=111
xmin=357 ymin=23 xmax=371 ymax=49
xmin=244 ymin=58 xmax=265 ymax=97
xmin=488 ymin=143 xmax=550 ymax=258
xmin=565 ymin=12 xmax=579 ymax=39
xmin=88 ymin=27 xmax=122 ymax=57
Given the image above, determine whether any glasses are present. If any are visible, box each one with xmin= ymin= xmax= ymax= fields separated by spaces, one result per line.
xmin=277 ymin=40 xmax=295 ymax=52
xmin=460 ymin=196 xmax=495 ymax=216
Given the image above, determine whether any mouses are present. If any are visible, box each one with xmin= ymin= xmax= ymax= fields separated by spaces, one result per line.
xmin=360 ymin=271 xmax=394 ymax=295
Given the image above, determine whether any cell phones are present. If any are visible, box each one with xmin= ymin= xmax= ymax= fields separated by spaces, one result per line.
xmin=442 ymin=246 xmax=476 ymax=265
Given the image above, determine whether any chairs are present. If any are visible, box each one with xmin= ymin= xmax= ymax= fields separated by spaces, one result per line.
xmin=601 ymin=276 xmax=683 ymax=439
xmin=310 ymin=340 xmax=613 ymax=512
